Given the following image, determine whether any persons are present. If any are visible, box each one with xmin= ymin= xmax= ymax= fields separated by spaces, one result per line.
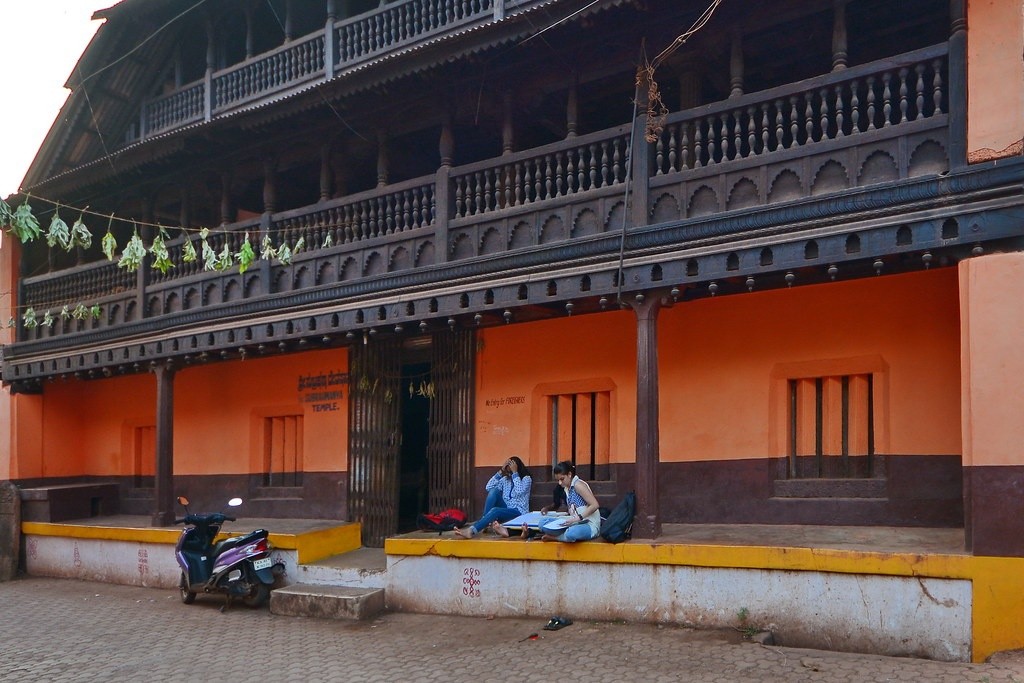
xmin=541 ymin=461 xmax=601 ymax=542
xmin=492 ymin=461 xmax=573 ymax=539
xmin=454 ymin=456 xmax=533 ymax=539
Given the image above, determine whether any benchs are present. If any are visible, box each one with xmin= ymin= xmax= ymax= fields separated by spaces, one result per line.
xmin=20 ymin=483 xmax=120 ymax=522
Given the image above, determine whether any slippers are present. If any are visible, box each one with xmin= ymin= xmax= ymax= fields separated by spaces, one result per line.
xmin=543 ymin=616 xmax=573 ymax=630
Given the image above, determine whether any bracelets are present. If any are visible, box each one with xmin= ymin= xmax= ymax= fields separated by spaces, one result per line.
xmin=500 ymin=468 xmax=503 ymax=472
xmin=578 ymin=514 xmax=583 ymax=521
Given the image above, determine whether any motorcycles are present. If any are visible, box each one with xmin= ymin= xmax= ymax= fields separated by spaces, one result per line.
xmin=170 ymin=496 xmax=285 ymax=612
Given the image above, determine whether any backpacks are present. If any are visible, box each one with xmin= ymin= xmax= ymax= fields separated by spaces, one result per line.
xmin=600 ymin=491 xmax=637 ymax=544
xmin=417 ymin=509 xmax=468 ymax=531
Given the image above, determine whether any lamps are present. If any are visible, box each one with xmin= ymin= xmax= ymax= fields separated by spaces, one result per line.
xmin=363 ymin=329 xmax=367 ymax=345
